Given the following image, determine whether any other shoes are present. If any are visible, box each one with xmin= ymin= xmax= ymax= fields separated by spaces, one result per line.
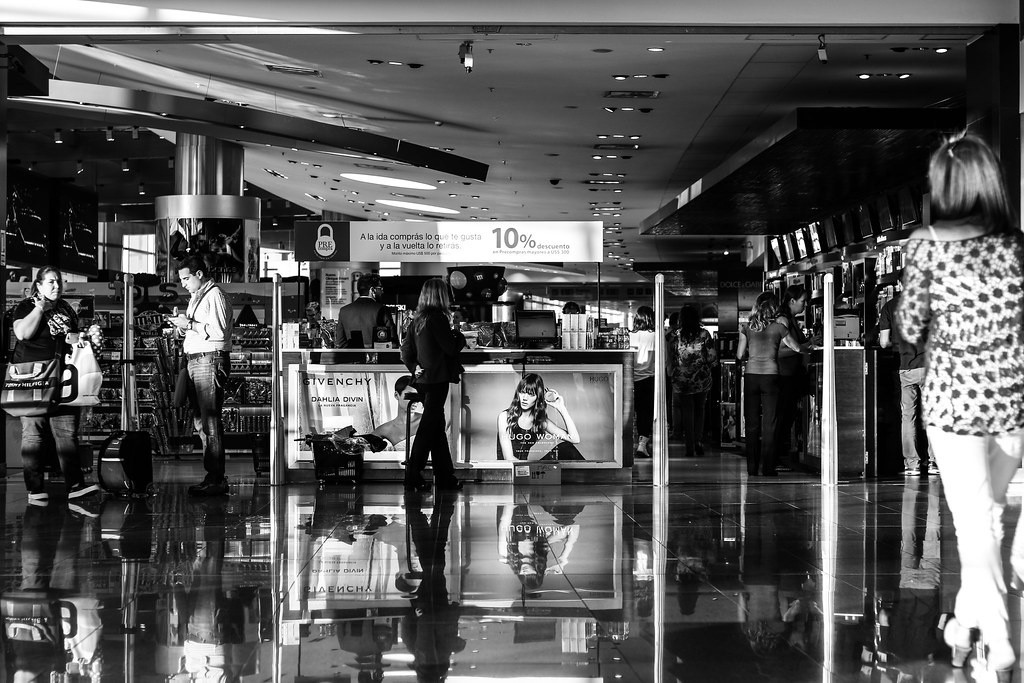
xmin=748 ymin=466 xmax=759 ymax=475
xmin=436 ymin=477 xmax=464 ymax=489
xmin=763 ymin=468 xmax=779 ymax=476
xmin=403 ymin=472 xmax=431 ymax=493
xmin=687 ymin=448 xmax=695 ymax=456
xmin=695 ymin=442 xmax=705 ymax=458
xmin=189 ymin=477 xmax=231 ymax=495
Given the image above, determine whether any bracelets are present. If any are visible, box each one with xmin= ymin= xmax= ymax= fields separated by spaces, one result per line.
xmin=808 ymin=342 xmax=810 ymax=344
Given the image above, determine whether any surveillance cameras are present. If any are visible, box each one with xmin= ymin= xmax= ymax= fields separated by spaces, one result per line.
xmin=550 ymin=180 xmax=559 ymax=185
xmin=459 ymin=44 xmax=467 ymax=64
xmin=818 ymin=48 xmax=828 ymax=64
xmin=465 ymin=54 xmax=473 ymax=74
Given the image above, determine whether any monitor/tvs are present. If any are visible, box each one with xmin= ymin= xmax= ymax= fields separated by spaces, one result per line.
xmin=514 ymin=309 xmax=558 ymax=344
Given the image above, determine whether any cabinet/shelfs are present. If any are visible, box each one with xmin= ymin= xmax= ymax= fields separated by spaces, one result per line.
xmin=4 ymin=291 xmax=275 ymax=460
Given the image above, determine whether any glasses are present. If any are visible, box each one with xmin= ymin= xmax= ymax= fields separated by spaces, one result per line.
xmin=374 ymin=286 xmax=383 ymax=291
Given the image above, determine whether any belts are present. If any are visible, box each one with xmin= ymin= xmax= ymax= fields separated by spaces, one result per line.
xmin=186 ymin=350 xmax=230 ymax=361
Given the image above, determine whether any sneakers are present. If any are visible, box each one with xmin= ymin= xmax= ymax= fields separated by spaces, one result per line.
xmin=904 ymin=459 xmax=921 ymax=476
xmin=68 ymin=484 xmax=99 ymax=499
xmin=28 ymin=490 xmax=49 ymax=501
xmin=928 ymin=459 xmax=940 ymax=475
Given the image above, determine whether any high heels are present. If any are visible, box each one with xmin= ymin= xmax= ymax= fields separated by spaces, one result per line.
xmin=935 ymin=612 xmax=972 ymax=668
xmin=981 ymin=646 xmax=1012 ymax=683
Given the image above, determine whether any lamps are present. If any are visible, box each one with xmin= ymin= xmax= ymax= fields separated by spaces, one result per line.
xmin=458 ymin=40 xmax=474 ymax=73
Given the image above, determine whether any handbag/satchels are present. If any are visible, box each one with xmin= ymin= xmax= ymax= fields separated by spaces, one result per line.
xmin=0 ymin=357 xmax=59 ymax=416
xmin=60 ymin=340 xmax=104 ymax=406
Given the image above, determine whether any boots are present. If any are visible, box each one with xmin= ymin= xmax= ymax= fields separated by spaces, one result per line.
xmin=636 ymin=435 xmax=650 ymax=457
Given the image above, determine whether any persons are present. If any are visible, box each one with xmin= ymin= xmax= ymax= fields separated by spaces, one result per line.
xmin=13 ymin=500 xmax=234 ymax=683
xmin=305 ymin=302 xmax=333 ymax=349
xmin=736 ymin=284 xmax=822 ymax=477
xmin=497 ymin=373 xmax=586 ymax=460
xmin=632 ymin=470 xmax=823 ymax=678
xmin=164 ymin=255 xmax=232 ymax=497
xmin=401 ymin=490 xmax=461 ymax=683
xmin=336 ymin=272 xmax=400 ymax=349
xmin=370 ymin=375 xmax=424 ymax=452
xmin=497 ymin=505 xmax=580 ymax=588
xmin=877 ymin=475 xmax=948 ymax=683
xmin=629 ymin=303 xmax=719 ymax=458
xmin=879 ymin=133 xmax=1024 ymax=683
xmin=400 ymin=278 xmax=467 ymax=493
xmin=562 ymin=302 xmax=580 ymax=314
xmin=12 ymin=264 xmax=99 ymax=500
